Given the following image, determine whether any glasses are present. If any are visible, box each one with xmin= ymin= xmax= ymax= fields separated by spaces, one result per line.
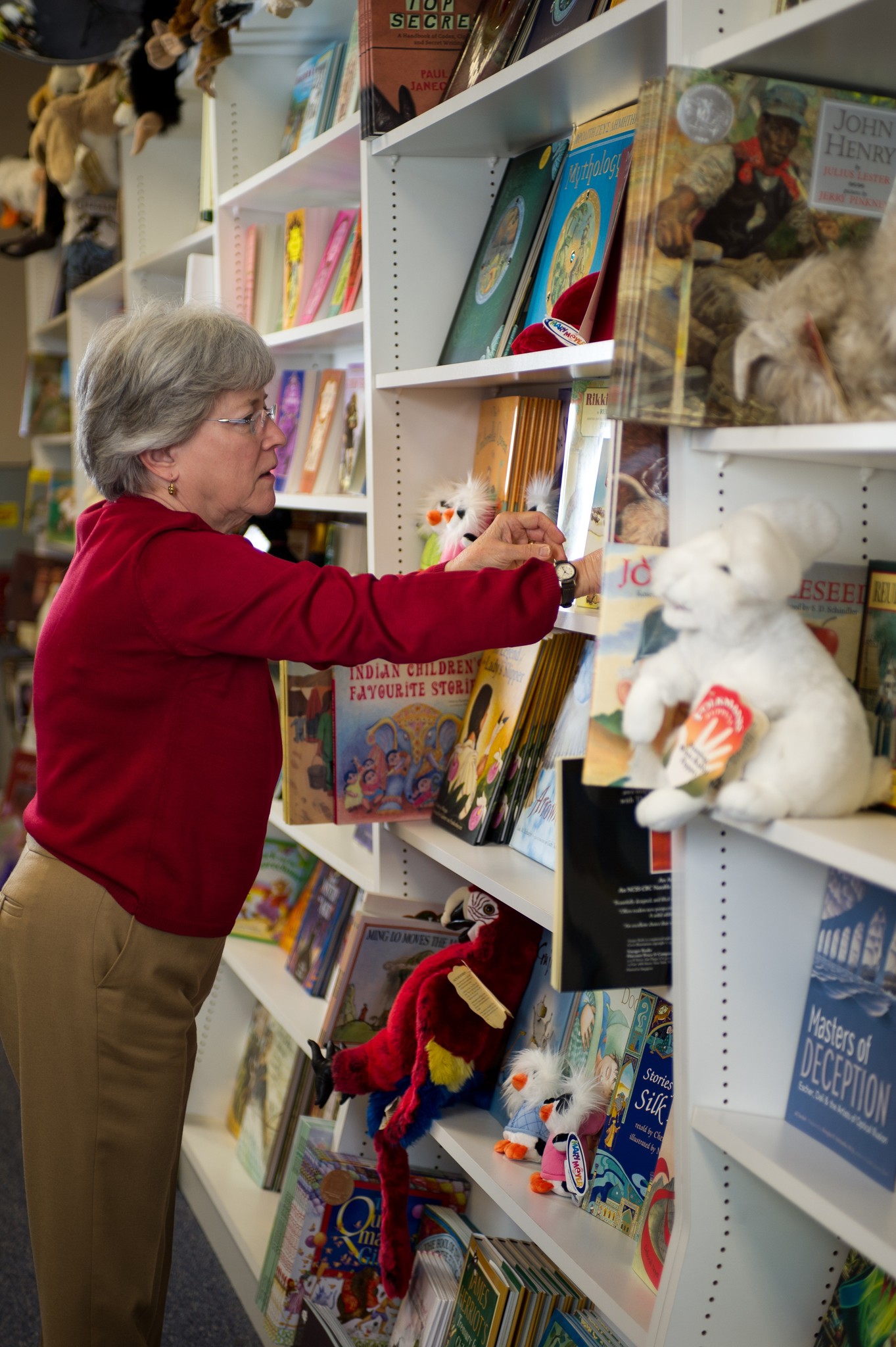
xmin=200 ymin=404 xmax=280 ymax=434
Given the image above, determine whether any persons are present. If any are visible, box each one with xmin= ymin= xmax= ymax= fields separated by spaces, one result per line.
xmin=1 ymin=301 xmax=603 ymax=1347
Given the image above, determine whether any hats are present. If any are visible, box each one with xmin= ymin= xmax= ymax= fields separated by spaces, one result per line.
xmin=759 ymin=89 xmax=809 ymax=125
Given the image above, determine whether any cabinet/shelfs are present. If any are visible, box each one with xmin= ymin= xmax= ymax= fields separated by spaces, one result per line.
xmin=23 ymin=0 xmax=895 ymax=1346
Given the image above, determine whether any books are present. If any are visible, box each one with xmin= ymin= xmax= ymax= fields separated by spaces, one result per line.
xmin=24 ymin=0 xmax=895 ymax=1347
